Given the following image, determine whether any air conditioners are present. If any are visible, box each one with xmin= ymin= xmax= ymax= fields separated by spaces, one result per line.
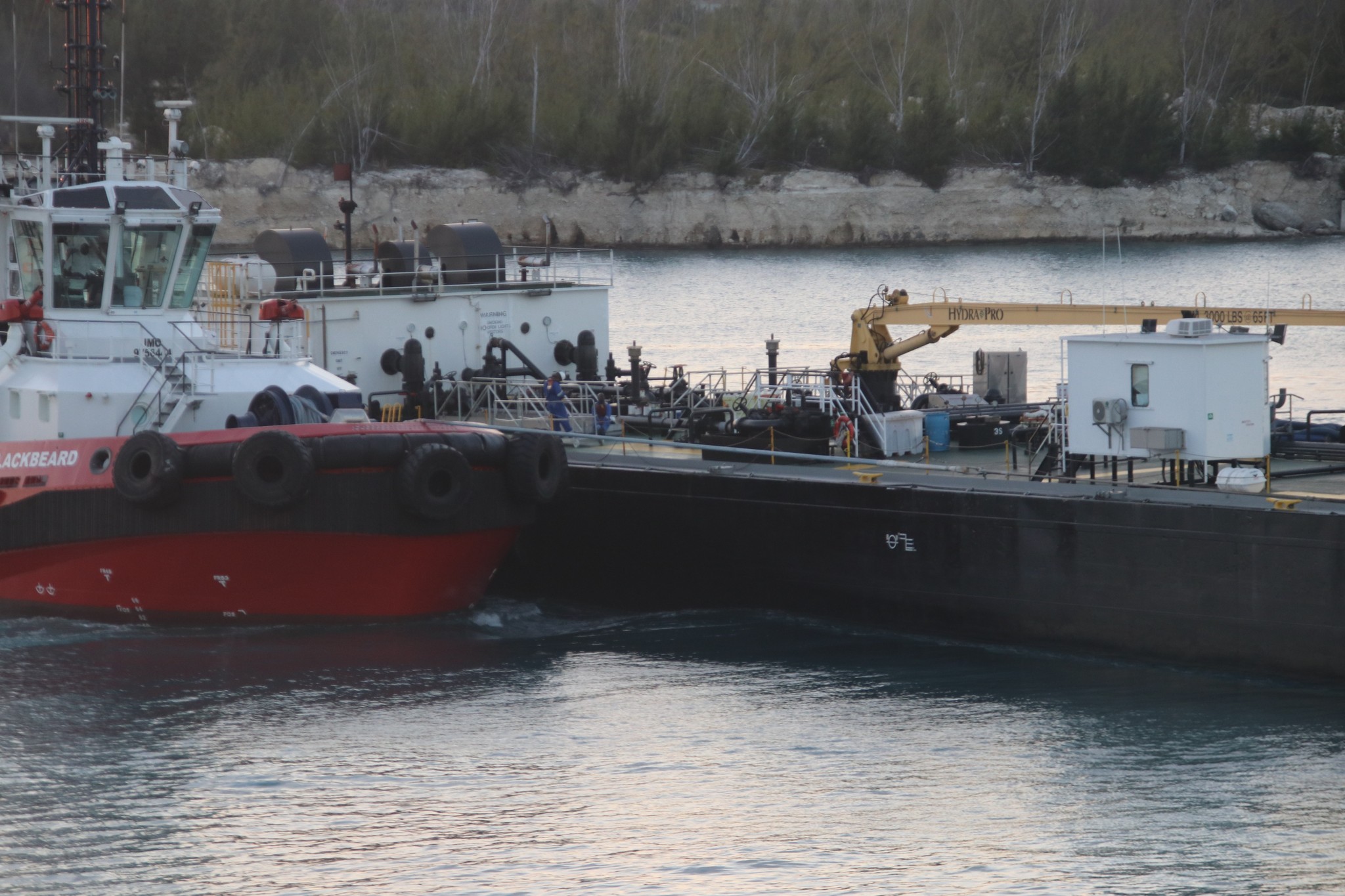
xmin=1093 ymin=398 xmax=1122 ymax=424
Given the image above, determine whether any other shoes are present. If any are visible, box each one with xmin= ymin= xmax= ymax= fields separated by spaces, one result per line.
xmin=599 ymin=439 xmax=605 ymax=445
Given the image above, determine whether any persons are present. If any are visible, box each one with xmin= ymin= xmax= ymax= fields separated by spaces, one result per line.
xmin=591 ymin=393 xmax=612 ymax=446
xmin=62 ymin=242 xmax=103 ymax=305
xmin=543 ymin=370 xmax=580 ymax=447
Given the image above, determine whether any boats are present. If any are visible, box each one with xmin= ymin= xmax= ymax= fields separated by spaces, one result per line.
xmin=1 ymin=100 xmax=568 ymax=648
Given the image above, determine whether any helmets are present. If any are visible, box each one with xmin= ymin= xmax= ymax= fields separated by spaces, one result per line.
xmin=558 ymin=371 xmax=566 ymax=381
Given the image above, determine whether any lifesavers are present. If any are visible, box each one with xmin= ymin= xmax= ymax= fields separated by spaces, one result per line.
xmin=506 ymin=430 xmax=568 ymax=508
xmin=395 ymin=443 xmax=474 ymax=520
xmin=34 ymin=321 xmax=54 ymax=350
xmin=231 ymin=430 xmax=313 ymax=510
xmin=112 ymin=431 xmax=183 ymax=505
xmin=833 ymin=415 xmax=855 ymax=448
